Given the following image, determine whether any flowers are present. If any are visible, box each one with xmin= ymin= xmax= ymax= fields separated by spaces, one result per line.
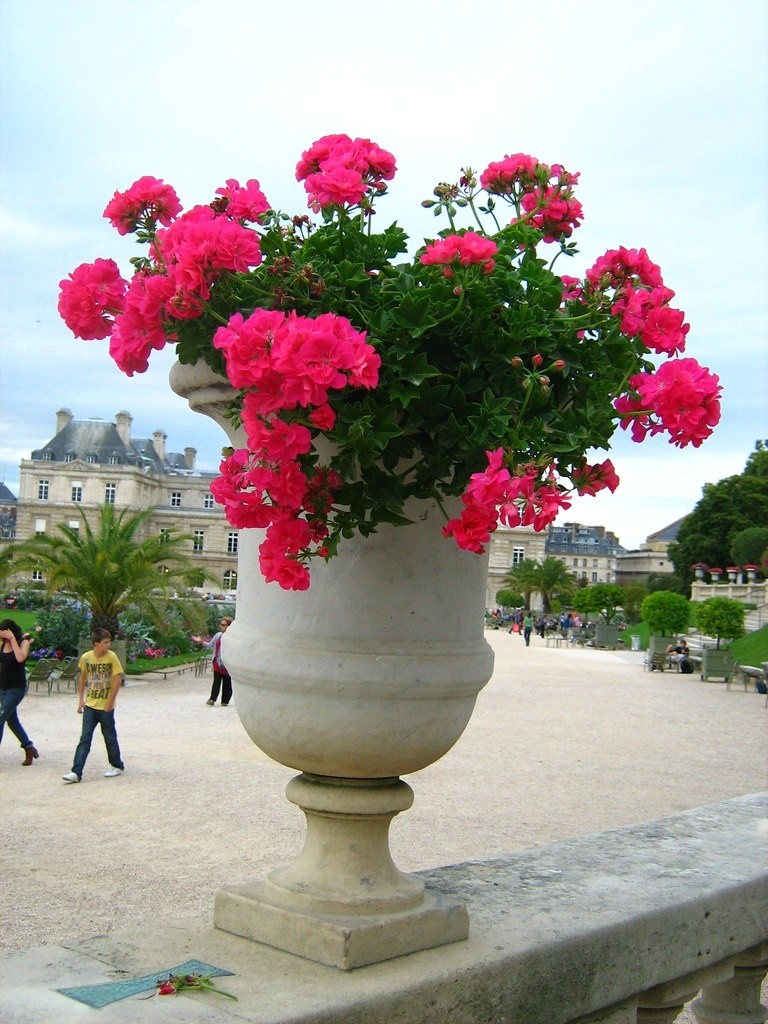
xmin=58 ymin=134 xmax=722 ymax=591
xmin=156 ymin=973 xmax=238 ymax=1001
xmin=744 ymin=564 xmax=759 ymax=570
xmin=726 ymin=567 xmax=740 ymax=574
xmin=690 ymin=562 xmax=723 ymax=573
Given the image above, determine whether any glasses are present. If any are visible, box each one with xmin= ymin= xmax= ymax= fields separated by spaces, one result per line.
xmin=219 ymin=622 xmax=229 ymax=626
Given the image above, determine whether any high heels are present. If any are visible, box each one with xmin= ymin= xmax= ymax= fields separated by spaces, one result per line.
xmin=22 ymin=746 xmax=39 ymax=766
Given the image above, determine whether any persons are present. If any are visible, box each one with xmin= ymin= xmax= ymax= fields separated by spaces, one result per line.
xmin=192 ymin=617 xmax=232 ymax=705
xmin=63 ymin=626 xmax=123 ymax=782
xmin=0 ymin=618 xmax=39 ymax=767
xmin=485 ymin=604 xmax=689 ymax=670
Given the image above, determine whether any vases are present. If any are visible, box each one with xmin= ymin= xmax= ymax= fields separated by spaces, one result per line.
xmin=726 ymin=570 xmax=738 ymax=585
xmin=692 ymin=567 xmax=707 ymax=585
xmin=710 ymin=572 xmax=720 ymax=585
xmin=746 ymin=568 xmax=756 ymax=585
xmin=167 ymin=352 xmax=496 ymax=970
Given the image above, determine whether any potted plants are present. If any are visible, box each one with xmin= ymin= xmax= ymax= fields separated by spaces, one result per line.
xmin=573 ymin=584 xmax=625 ymax=650
xmin=640 ymin=591 xmax=691 ymax=665
xmin=0 ymin=502 xmax=224 ymax=686
xmin=694 ymin=597 xmax=746 ymax=683
xmin=496 ymin=553 xmax=579 ymax=623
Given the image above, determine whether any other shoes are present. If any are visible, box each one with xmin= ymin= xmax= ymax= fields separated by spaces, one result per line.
xmin=206 ymin=700 xmax=214 ymax=705
xmin=222 ymin=703 xmax=227 ymax=706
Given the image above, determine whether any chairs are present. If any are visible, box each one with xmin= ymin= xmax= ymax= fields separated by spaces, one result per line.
xmin=644 ymin=652 xmax=668 ymax=672
xmin=546 ymin=628 xmax=586 ymax=648
xmin=26 ymin=656 xmax=81 ymax=696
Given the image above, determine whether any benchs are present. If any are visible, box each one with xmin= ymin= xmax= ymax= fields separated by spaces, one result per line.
xmin=727 ymin=673 xmax=768 ymax=709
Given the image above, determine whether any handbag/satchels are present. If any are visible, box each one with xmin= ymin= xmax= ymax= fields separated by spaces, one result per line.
xmin=513 ymin=623 xmax=519 ymax=632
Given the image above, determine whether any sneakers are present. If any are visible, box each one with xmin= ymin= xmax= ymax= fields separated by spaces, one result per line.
xmin=104 ymin=765 xmax=123 ymax=776
xmin=63 ymin=772 xmax=79 ymax=782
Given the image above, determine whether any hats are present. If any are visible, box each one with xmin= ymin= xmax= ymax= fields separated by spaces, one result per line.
xmin=761 ymin=661 xmax=768 ymax=670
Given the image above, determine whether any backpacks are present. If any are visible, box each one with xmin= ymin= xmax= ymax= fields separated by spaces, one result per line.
xmin=681 ymin=660 xmax=693 ymax=673
xmin=757 ymin=682 xmax=768 ymax=694
